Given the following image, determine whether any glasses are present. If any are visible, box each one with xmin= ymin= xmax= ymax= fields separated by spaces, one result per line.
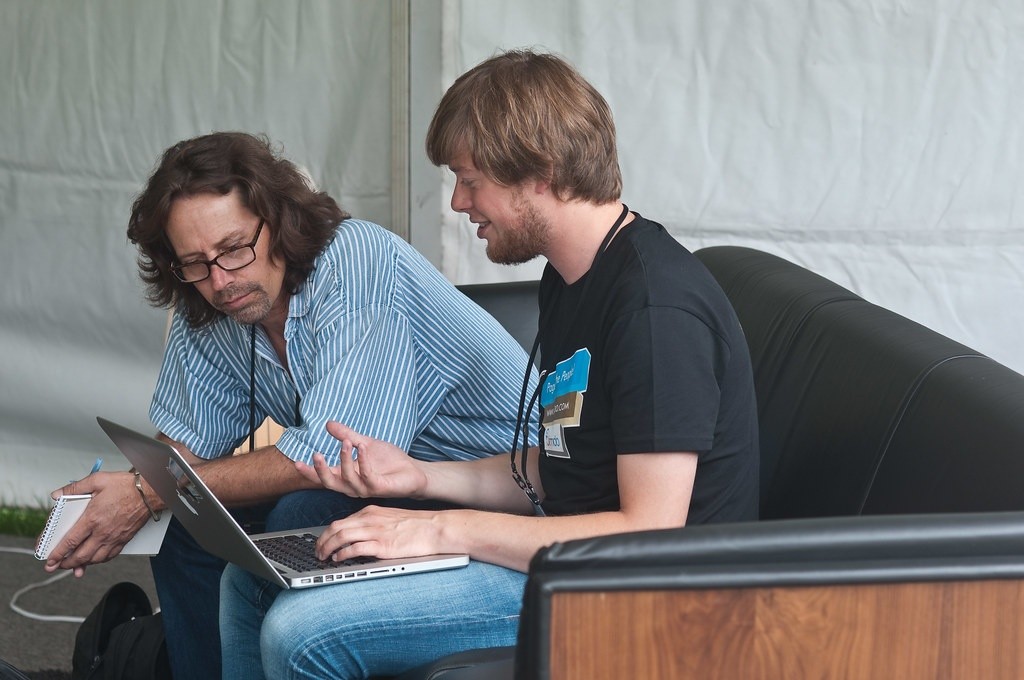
xmin=168 ymin=216 xmax=266 ymax=284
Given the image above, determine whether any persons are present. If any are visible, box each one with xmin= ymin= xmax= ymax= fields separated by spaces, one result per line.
xmin=38 ymin=133 xmax=540 ymax=680
xmin=219 ymin=50 xmax=760 ymax=680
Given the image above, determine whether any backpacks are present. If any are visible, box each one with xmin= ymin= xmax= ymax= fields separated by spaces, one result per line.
xmin=72 ymin=582 xmax=172 ymax=680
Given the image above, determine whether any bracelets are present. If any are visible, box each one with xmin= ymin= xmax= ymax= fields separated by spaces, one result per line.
xmin=134 ymin=470 xmax=161 ymax=522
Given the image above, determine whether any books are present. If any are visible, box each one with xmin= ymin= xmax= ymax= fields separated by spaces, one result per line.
xmin=34 ymin=494 xmax=173 ymax=560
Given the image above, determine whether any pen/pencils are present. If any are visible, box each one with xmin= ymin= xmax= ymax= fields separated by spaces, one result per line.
xmin=90 ymin=457 xmax=104 ymax=474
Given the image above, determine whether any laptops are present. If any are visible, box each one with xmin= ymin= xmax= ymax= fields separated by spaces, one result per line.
xmin=96 ymin=416 xmax=470 ymax=588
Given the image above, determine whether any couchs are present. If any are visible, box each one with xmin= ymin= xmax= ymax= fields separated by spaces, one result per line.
xmin=414 ymin=241 xmax=1024 ymax=680
